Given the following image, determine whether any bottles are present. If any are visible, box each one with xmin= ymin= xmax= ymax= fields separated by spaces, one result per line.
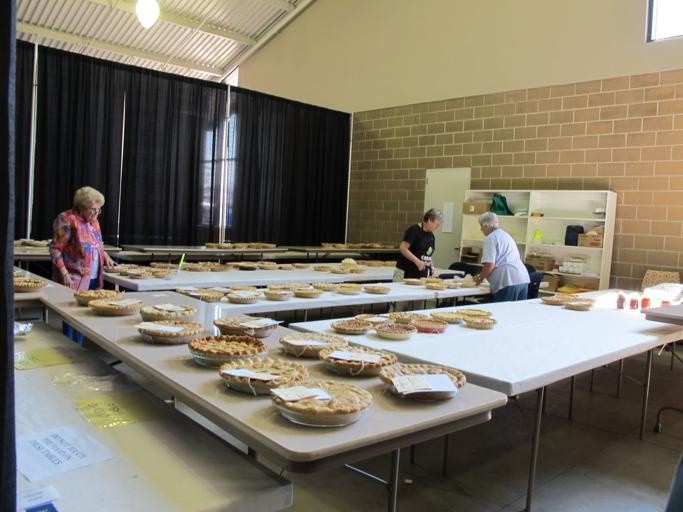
xmin=617 ymin=290 xmax=671 ymax=315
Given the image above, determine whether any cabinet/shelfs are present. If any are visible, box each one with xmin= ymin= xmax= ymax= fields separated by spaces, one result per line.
xmin=460 ymin=189 xmax=617 ymax=292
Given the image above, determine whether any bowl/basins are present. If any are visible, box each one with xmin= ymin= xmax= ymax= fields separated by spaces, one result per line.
xmin=377 ymin=331 xmax=414 ymax=340
xmin=282 ymin=348 xmax=329 ymax=361
xmin=362 ymin=315 xmax=469 ymax=334
xmin=187 ymin=352 xmax=266 ymax=364
xmin=270 ymin=403 xmax=375 ymax=428
xmin=377 ymin=378 xmax=466 ymax=397
xmin=142 ymin=315 xmax=197 ymax=321
xmin=318 ymin=360 xmax=392 ymax=379
xmin=218 ymin=327 xmax=275 ymax=336
xmin=334 ymin=329 xmax=369 ymax=334
xmin=224 ymin=382 xmax=276 ymax=399
xmin=138 ymin=333 xmax=202 ymax=345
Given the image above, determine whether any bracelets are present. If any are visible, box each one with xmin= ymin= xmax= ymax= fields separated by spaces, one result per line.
xmin=477 ymin=274 xmax=482 ymax=283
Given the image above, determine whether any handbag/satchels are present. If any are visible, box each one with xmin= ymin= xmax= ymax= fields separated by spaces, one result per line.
xmin=396 ymin=254 xmax=407 ymax=270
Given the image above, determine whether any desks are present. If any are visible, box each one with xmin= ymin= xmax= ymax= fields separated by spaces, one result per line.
xmin=11 ymin=244 xmax=683 ymax=512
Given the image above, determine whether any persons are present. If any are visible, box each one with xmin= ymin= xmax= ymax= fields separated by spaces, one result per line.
xmin=49 ymin=186 xmax=119 ymax=346
xmin=473 ymin=211 xmax=531 ymax=302
xmin=392 ymin=208 xmax=445 ymax=282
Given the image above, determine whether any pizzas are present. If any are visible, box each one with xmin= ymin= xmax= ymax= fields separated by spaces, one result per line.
xmin=104 ymin=258 xmax=494 ymax=304
xmin=14 ymin=277 xmax=45 ymax=291
xmin=219 ymin=357 xmax=373 ymax=427
xmin=73 ymin=287 xmax=142 ymax=316
xmin=138 ymin=305 xmax=203 ymax=344
xmin=331 ymin=306 xmax=494 ymax=340
xmin=188 ymin=314 xmax=278 ymax=368
xmin=279 ymin=332 xmax=466 ymax=402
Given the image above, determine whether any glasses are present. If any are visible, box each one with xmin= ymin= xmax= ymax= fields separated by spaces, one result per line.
xmin=88 ymin=207 xmax=102 ymax=214
